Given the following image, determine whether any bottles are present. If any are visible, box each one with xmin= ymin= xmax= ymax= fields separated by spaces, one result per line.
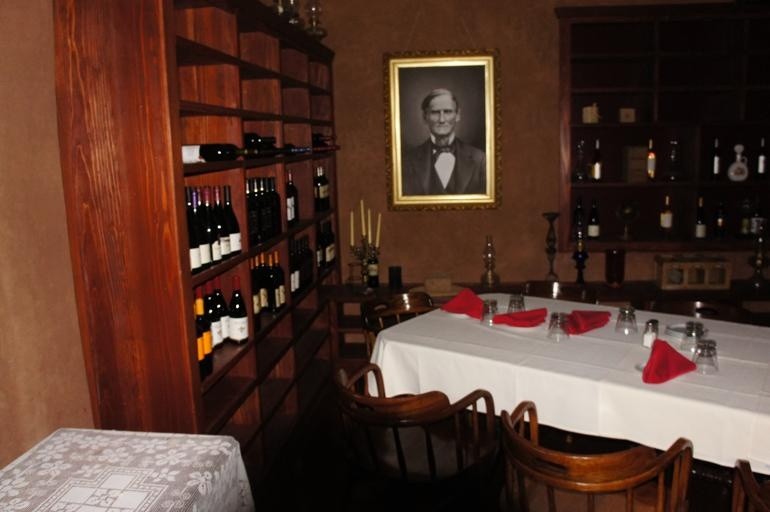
xmin=642 ymin=321 xmax=657 ymax=347
xmin=574 ymin=139 xmax=603 ymax=183
xmin=244 ymin=129 xmax=342 ymax=163
xmin=181 ymin=168 xmax=337 ymax=381
xmin=572 ymin=196 xmax=602 ymax=240
xmin=646 ymin=137 xmax=769 ymax=239
xmin=480 ymin=236 xmax=501 ymax=289
xmin=368 ymin=250 xmax=378 ymax=288
xmin=180 ymin=141 xmax=258 ymax=164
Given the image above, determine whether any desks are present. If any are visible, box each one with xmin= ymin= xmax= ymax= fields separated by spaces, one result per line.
xmin=0 ymin=422 xmax=260 ymax=511
xmin=364 ymin=288 xmax=769 ymax=499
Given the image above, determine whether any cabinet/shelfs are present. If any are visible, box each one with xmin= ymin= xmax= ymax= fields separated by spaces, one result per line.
xmin=551 ymin=1 xmax=770 ymax=260
xmin=47 ymin=1 xmax=346 ymax=510
xmin=311 ymin=276 xmax=770 ymax=408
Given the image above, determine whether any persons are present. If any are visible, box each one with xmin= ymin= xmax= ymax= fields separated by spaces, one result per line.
xmin=403 ymin=89 xmax=486 ymax=196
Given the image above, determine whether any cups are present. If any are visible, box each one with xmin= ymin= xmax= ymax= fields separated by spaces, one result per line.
xmin=506 ymin=297 xmax=525 ymax=316
xmin=616 ymin=305 xmax=638 ymax=337
xmin=581 ymin=107 xmax=598 ymax=124
xmin=680 ymin=322 xmax=705 ymax=353
xmin=481 ymin=300 xmax=500 ymax=325
xmin=690 ymin=339 xmax=719 ymax=375
xmin=388 ymin=266 xmax=402 ymax=288
xmin=348 ymin=263 xmax=363 ymax=286
xmin=546 ymin=312 xmax=570 ymax=343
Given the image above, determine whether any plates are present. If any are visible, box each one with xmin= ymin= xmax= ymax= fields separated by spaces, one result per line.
xmin=665 ymin=323 xmax=710 ymax=339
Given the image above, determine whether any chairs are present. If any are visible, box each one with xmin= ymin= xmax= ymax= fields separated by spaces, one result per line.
xmin=356 ymin=285 xmax=437 ymax=363
xmin=726 ymin=453 xmax=769 ymax=511
xmin=336 ymin=355 xmax=511 ymax=511
xmin=494 ymin=396 xmax=695 ymax=512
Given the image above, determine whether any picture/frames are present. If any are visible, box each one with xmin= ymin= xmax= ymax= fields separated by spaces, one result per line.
xmin=377 ymin=44 xmax=506 ymax=217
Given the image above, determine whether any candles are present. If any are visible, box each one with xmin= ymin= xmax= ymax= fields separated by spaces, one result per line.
xmin=344 ymin=195 xmax=385 ymax=252
